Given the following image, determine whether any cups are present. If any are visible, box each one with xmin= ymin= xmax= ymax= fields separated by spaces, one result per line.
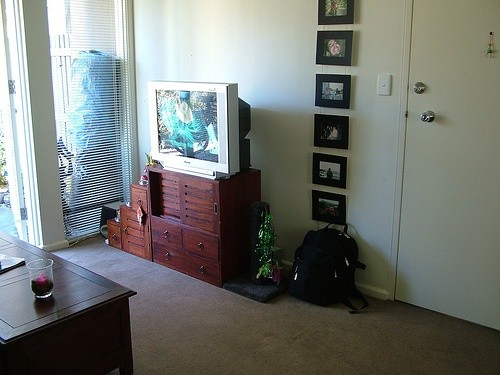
xmin=26 ymin=258 xmax=54 ymax=299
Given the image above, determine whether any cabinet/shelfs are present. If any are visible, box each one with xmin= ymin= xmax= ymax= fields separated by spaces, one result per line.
xmin=107 ymin=163 xmax=262 ymax=288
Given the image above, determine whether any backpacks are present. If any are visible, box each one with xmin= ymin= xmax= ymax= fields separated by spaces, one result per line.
xmin=288 ymin=222 xmax=369 ymax=314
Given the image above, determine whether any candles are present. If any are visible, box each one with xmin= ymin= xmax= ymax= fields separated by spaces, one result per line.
xmin=36 ymin=272 xmax=48 ymax=286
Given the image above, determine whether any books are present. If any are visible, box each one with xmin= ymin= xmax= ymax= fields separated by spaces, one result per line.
xmin=0 ymin=254 xmax=26 ymax=275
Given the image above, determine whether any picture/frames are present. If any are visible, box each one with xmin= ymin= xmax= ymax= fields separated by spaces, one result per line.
xmin=312 ymin=152 xmax=347 ymax=189
xmin=312 ymin=189 xmax=346 ymax=226
xmin=316 ymin=30 xmax=354 ymax=66
xmin=318 ymin=0 xmax=354 ymax=25
xmin=315 ymin=74 xmax=351 ymax=109
xmin=314 ymin=114 xmax=349 ymax=150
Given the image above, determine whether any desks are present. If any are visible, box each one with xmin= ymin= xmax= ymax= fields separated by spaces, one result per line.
xmin=0 ymin=229 xmax=137 ymax=375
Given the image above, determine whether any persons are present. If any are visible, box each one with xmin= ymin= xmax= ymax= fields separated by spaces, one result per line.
xmin=325 ymin=87 xmax=340 ymax=96
xmin=321 ymin=205 xmax=334 ymax=217
xmin=330 ymin=0 xmax=338 ymax=17
xmin=322 ymin=126 xmax=339 ymax=140
xmin=327 ymin=168 xmax=333 ymax=179
xmin=326 ymin=39 xmax=341 ymax=57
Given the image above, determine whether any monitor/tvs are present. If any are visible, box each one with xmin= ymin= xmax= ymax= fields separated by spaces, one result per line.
xmin=147 ymin=80 xmax=252 ymax=180
